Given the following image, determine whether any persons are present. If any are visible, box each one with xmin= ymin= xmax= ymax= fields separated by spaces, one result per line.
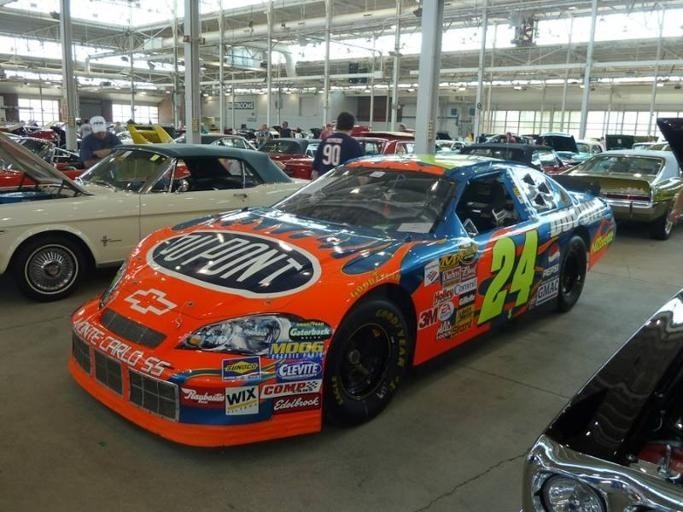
xmin=256 ymin=120 xmax=304 ymax=147
xmin=311 ymin=111 xmax=367 ymax=181
xmin=79 ymin=117 xmax=124 ymax=168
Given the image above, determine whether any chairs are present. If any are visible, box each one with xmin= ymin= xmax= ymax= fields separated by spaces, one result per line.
xmin=372 ymin=174 xmax=509 ymax=228
xmin=195 ymin=174 xmax=247 ymax=189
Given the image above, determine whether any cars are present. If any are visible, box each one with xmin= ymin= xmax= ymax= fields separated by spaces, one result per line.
xmin=516 ymin=116 xmax=683 ymax=511
xmin=558 ymin=148 xmax=683 ymax=241
xmin=0 ymin=131 xmax=313 ymax=303
xmin=66 ymin=152 xmax=617 ymax=450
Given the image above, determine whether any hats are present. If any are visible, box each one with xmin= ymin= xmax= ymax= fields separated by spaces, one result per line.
xmin=90 ymin=116 xmax=107 ymax=134
